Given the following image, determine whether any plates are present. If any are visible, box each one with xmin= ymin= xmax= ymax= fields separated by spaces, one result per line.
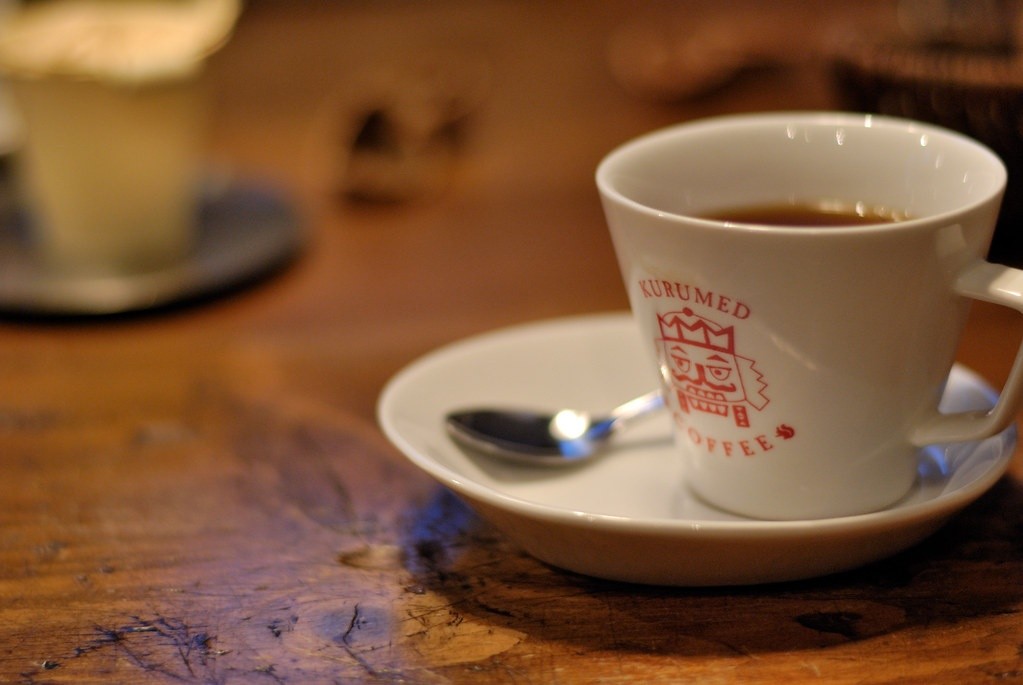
xmin=375 ymin=311 xmax=1023 ymax=588
xmin=0 ymin=157 xmax=301 ymax=313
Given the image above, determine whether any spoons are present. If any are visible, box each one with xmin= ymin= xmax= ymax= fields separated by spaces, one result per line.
xmin=444 ymin=387 xmax=668 ymax=467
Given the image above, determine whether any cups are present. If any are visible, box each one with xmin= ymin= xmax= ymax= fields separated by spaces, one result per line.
xmin=0 ymin=0 xmax=244 ymax=269
xmin=596 ymin=111 xmax=1023 ymax=520
